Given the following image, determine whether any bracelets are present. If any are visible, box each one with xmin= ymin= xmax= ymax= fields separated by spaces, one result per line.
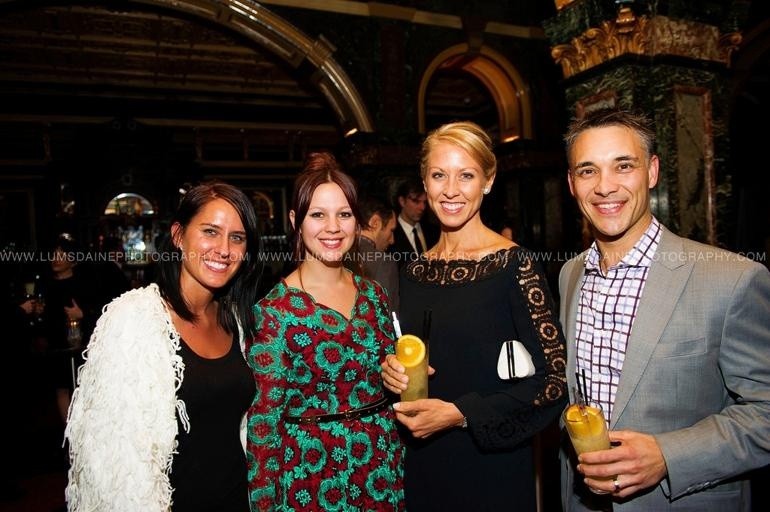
xmin=459 ymin=414 xmax=470 ymax=430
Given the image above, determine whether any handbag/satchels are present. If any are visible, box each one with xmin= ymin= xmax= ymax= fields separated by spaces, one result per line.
xmin=497 ymin=340 xmax=535 ymax=380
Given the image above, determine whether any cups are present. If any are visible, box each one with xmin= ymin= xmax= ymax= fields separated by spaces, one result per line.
xmin=562 ymin=401 xmax=616 ymax=495
xmin=394 ymin=340 xmax=429 ymax=417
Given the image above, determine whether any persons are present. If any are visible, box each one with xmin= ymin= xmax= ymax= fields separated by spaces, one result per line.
xmin=559 ymin=104 xmax=767 ymax=510
xmin=392 ymin=174 xmax=439 ymax=261
xmin=0 ymin=230 xmax=173 ymax=512
xmin=250 ymin=151 xmax=411 ymax=512
xmin=380 ymin=119 xmax=569 ymax=510
xmin=69 ymin=179 xmax=263 ymax=511
xmin=352 ymin=191 xmax=402 ymax=312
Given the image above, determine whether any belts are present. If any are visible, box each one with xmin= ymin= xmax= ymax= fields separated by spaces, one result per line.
xmin=285 ymin=398 xmax=389 ymax=423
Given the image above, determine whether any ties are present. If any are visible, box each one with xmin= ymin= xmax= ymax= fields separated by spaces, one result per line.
xmin=413 ymin=228 xmax=423 ymax=257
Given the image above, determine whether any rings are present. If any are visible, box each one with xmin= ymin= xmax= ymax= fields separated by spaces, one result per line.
xmin=613 ymin=475 xmax=621 ymax=494
